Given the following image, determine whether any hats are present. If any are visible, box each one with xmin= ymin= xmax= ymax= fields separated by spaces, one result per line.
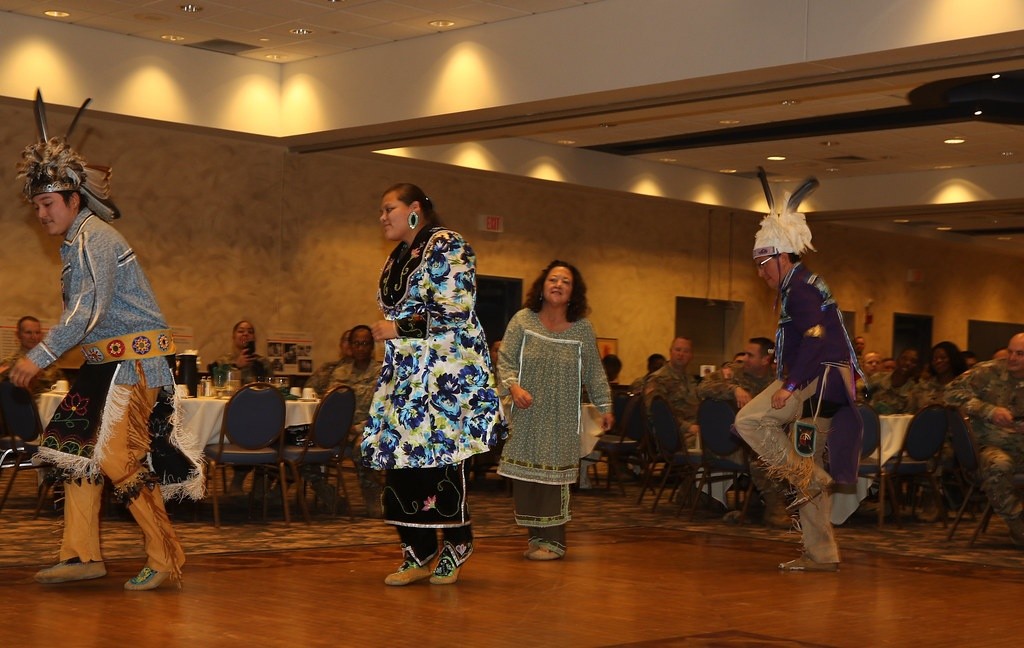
xmin=751 ymin=166 xmax=819 ymax=259
xmin=13 ymin=88 xmax=121 ymax=224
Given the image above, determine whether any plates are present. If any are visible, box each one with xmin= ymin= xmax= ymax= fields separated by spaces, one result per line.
xmin=299 ymin=398 xmax=320 ymax=402
xmin=53 ymin=391 xmax=68 ymax=395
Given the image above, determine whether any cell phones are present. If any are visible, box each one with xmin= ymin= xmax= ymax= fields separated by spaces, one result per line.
xmin=243 ymin=339 xmax=255 ymax=356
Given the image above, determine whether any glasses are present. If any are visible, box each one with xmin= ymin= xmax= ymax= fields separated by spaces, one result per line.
xmin=754 ymin=257 xmax=775 ymax=272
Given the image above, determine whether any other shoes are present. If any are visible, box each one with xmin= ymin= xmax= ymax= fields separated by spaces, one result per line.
xmin=124 ymin=566 xmax=172 ymax=591
xmin=778 ymin=556 xmax=840 ymax=574
xmin=783 ymin=474 xmax=834 ymax=515
xmin=430 ymin=549 xmax=463 ymax=585
xmin=523 ymin=539 xmax=540 ymax=557
xmin=529 ymin=543 xmax=567 ymax=561
xmin=33 ymin=559 xmax=107 ymax=584
xmin=384 ymin=558 xmax=431 ymax=585
xmin=31 ymin=475 xmax=382 ymax=531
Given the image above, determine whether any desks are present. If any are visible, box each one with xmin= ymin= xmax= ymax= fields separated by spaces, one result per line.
xmin=695 ymin=414 xmax=916 ymax=526
xmin=500 ymin=403 xmax=603 ymax=497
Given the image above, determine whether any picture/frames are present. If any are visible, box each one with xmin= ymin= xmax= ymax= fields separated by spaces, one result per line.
xmin=596 ymin=338 xmax=618 ymax=360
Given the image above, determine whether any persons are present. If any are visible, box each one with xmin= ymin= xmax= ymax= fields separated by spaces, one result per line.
xmin=497 ymin=260 xmax=614 ymax=560
xmin=11 ymin=139 xmax=205 ymax=589
xmin=734 ymin=208 xmax=867 ymax=570
xmin=464 ymin=340 xmax=502 ymax=485
xmin=630 ymin=335 xmax=798 ymax=530
xmin=853 ymin=333 xmax=1024 ymax=544
xmin=242 ymin=324 xmax=383 ymax=508
xmin=361 ymin=183 xmax=513 ymax=584
xmin=603 ymin=354 xmax=621 ymax=381
xmin=0 ymin=316 xmax=59 ymax=503
xmin=214 ymin=321 xmax=273 ymax=497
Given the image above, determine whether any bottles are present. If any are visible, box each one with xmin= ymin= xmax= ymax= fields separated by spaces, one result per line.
xmin=200 ymin=376 xmax=212 ymax=396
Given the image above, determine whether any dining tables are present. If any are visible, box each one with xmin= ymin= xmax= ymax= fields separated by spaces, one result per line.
xmin=39 ymin=391 xmax=321 ymax=522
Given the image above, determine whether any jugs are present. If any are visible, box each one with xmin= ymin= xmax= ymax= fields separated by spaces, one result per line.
xmin=176 ymin=355 xmax=197 ymax=398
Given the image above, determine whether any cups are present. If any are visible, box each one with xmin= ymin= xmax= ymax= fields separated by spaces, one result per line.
xmin=302 ymin=388 xmax=316 ymax=399
xmin=289 ymin=387 xmax=301 ymax=397
xmin=51 ymin=381 xmax=68 ymax=392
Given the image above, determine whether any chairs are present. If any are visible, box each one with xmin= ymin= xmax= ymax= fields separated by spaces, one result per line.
xmin=193 ymin=382 xmax=290 ymax=528
xmin=576 ymin=378 xmax=994 ymax=548
xmin=259 ymin=384 xmax=356 ymax=525
xmin=0 ymin=380 xmax=61 ymax=520
